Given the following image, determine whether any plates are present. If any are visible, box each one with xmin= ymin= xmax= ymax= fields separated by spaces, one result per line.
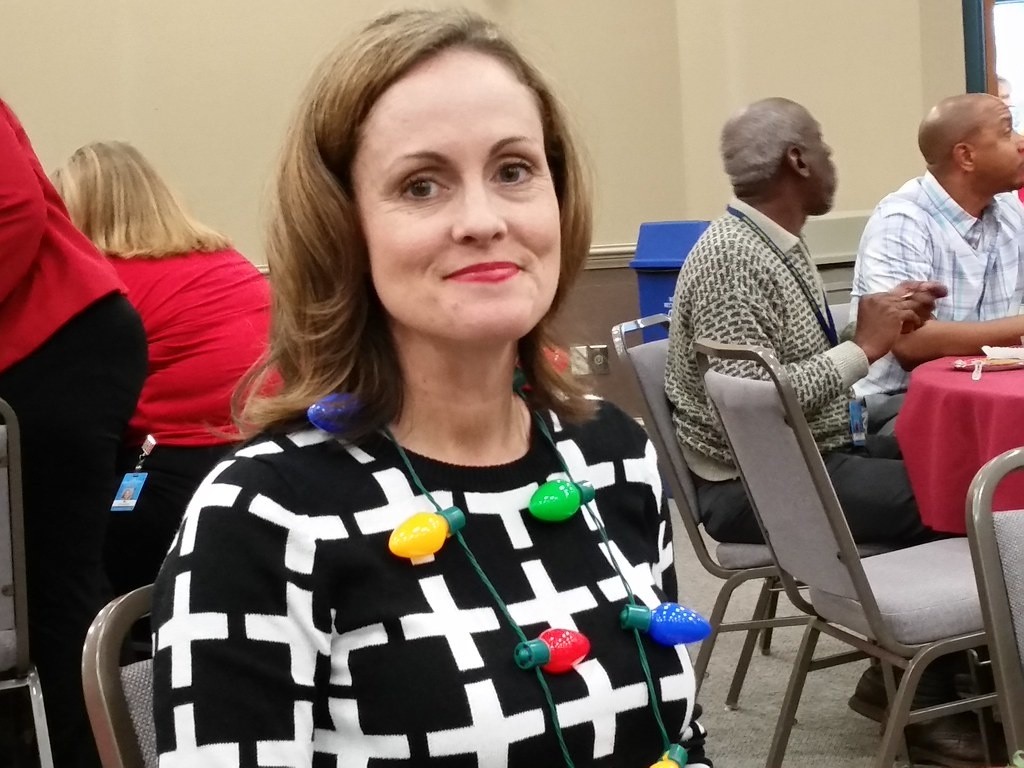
xmin=954 ymin=357 xmax=1024 ymax=371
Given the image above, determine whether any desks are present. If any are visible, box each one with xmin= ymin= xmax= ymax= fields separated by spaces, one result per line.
xmin=893 ymin=352 xmax=1024 ymax=662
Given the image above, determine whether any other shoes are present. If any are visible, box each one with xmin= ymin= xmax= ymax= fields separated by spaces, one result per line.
xmin=878 ymin=693 xmax=1010 ymax=768
xmin=847 ymin=662 xmax=889 ymax=723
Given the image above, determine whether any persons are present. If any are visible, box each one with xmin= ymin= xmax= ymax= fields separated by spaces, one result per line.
xmin=661 ymin=99 xmax=1009 ymax=767
xmin=153 ymin=6 xmax=712 ymax=767
xmin=122 ymin=489 xmax=131 ymax=500
xmin=49 ymin=141 xmax=282 ymax=593
xmin=849 ymin=93 xmax=1024 ymax=438
xmin=0 ymin=93 xmax=148 ymax=768
xmin=998 ymin=78 xmax=1024 ymax=204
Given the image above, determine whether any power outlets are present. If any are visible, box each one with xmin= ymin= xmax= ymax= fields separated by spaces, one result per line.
xmin=569 ymin=344 xmax=609 ymax=377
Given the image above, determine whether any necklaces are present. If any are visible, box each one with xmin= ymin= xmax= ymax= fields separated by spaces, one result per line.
xmin=307 ymin=348 xmax=711 ymax=768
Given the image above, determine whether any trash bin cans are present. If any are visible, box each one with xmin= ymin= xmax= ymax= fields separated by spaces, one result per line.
xmin=628 ymin=220 xmax=714 ymax=344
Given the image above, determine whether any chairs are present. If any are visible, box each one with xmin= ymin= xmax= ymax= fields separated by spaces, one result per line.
xmin=81 ymin=581 xmax=158 ymax=768
xmin=612 ymin=279 xmax=1024 ymax=768
xmin=0 ymin=400 xmax=56 ymax=768
